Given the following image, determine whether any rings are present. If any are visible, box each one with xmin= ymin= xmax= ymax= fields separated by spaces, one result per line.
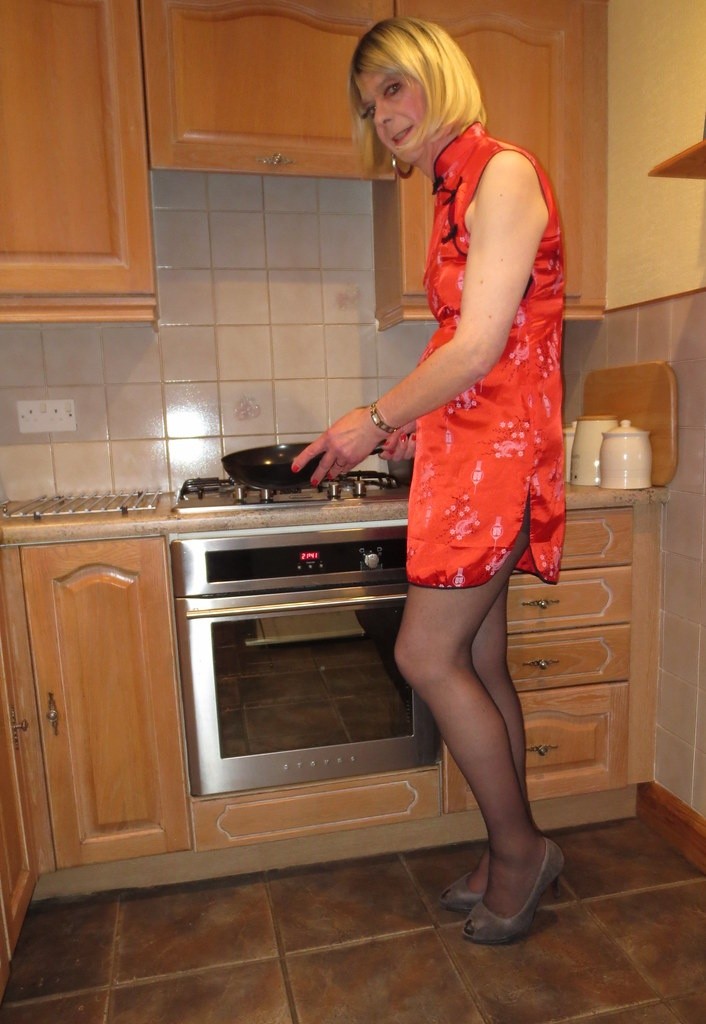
xmin=335 ymin=460 xmax=343 ymax=468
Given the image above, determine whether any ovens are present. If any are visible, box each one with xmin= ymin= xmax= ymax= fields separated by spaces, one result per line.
xmin=175 ymin=524 xmax=438 ymax=793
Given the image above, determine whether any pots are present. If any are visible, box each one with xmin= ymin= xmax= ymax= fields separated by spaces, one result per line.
xmin=221 ymin=437 xmax=387 ymax=490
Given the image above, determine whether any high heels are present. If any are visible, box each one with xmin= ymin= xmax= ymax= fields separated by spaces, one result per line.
xmin=438 ymin=837 xmax=566 ymax=945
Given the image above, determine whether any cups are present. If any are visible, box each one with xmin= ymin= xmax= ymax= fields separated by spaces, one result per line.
xmin=570 ymin=418 xmax=619 ymax=486
xmin=560 ymin=421 xmax=578 ymax=484
xmin=599 ymin=421 xmax=652 ymax=490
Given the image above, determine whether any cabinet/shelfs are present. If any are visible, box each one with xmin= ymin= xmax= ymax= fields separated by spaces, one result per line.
xmin=443 ymin=505 xmax=636 ymax=811
xmin=0 ymin=549 xmax=40 ymax=954
xmin=138 ymin=1 xmax=395 ymax=181
xmin=20 ymin=537 xmax=190 ymax=873
xmin=373 ymin=0 xmax=607 ymax=334
xmin=0 ymin=0 xmax=160 ymax=326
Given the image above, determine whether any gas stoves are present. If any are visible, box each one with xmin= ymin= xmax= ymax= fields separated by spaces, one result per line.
xmin=182 ymin=472 xmax=403 ymax=508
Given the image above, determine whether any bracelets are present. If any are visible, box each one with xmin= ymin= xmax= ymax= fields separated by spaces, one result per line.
xmin=370 ymin=399 xmax=398 ymax=433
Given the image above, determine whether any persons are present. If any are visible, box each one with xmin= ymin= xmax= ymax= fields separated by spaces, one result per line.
xmin=289 ymin=16 xmax=579 ymax=948
